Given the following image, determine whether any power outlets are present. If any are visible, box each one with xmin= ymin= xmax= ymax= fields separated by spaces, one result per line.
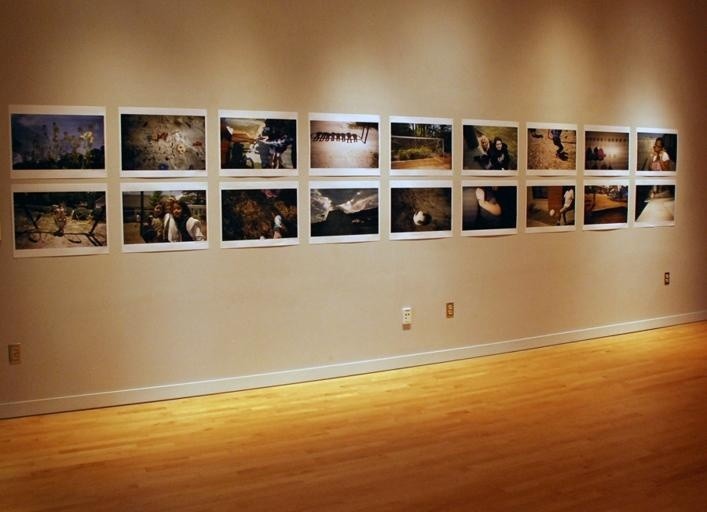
xmin=445 ymin=302 xmax=455 ymax=319
xmin=9 ymin=342 xmax=22 ymax=365
xmin=664 ymin=272 xmax=669 ymax=285
xmin=402 ymin=306 xmax=413 ymax=325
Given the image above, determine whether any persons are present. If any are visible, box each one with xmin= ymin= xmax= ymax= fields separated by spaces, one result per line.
xmin=556 ymin=186 xmax=574 ymax=226
xmin=547 ymin=129 xmax=564 ymax=156
xmin=141 ymin=202 xmax=165 ymax=242
xmin=313 ymin=131 xmax=359 ymax=143
xmin=271 ymin=209 xmax=287 ymax=239
xmin=649 ymin=137 xmax=671 ymax=172
xmin=479 ymin=136 xmax=490 ymax=156
xmin=171 ymin=198 xmax=205 ymax=243
xmin=53 ymin=204 xmax=67 ymax=234
xmin=487 ymin=136 xmax=510 ymax=170
